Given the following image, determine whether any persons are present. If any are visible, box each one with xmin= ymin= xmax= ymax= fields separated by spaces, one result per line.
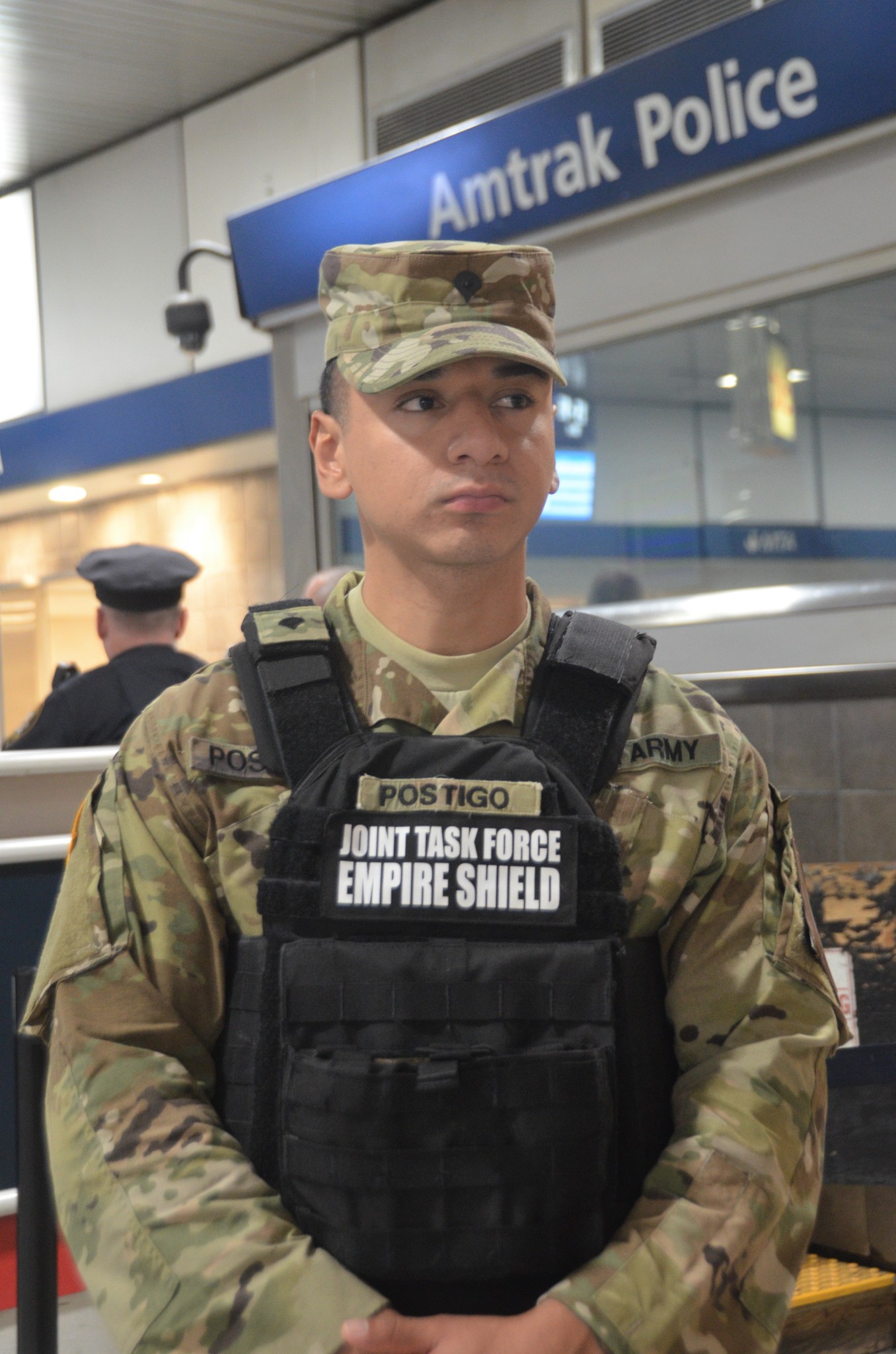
xmin=23 ymin=239 xmax=844 ymax=1354
xmin=2 ymin=543 xmax=212 ymax=750
xmin=306 ymin=566 xmax=351 ymax=607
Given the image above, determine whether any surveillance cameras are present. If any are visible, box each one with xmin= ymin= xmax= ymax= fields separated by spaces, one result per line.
xmin=163 ymin=297 xmax=213 ymax=354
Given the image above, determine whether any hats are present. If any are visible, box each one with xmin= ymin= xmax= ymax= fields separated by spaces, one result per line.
xmin=316 ymin=240 xmax=568 ymax=386
xmin=78 ymin=545 xmax=200 ymax=611
